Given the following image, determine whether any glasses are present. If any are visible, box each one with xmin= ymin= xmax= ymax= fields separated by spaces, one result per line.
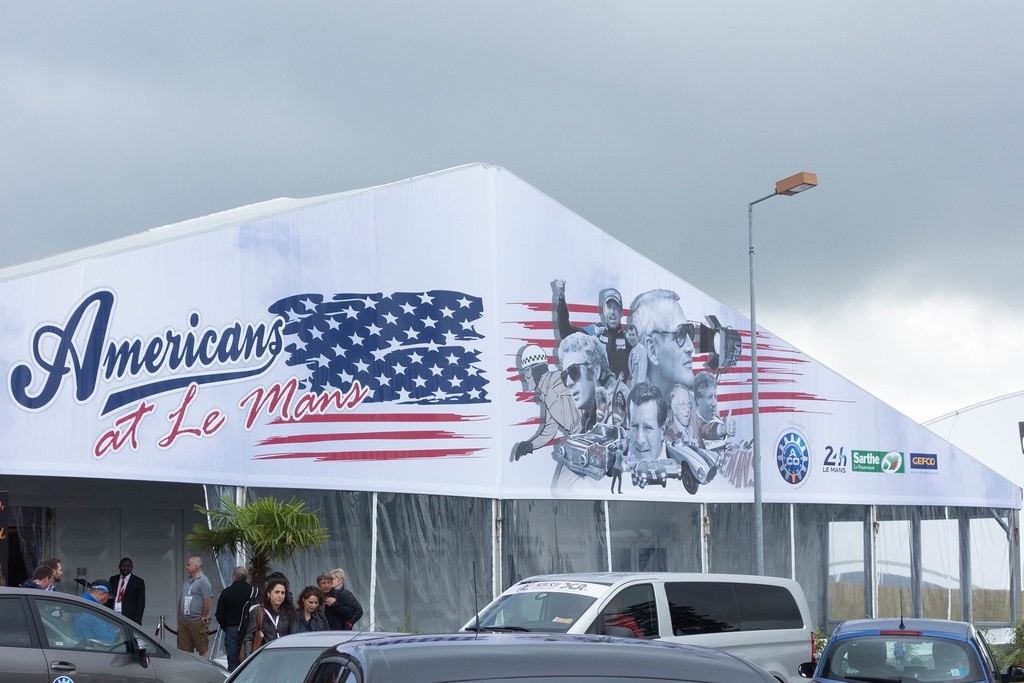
xmin=560 ymin=363 xmax=589 ymax=387
xmin=679 ymin=400 xmax=692 ymax=409
xmin=649 ymin=324 xmax=696 ymax=347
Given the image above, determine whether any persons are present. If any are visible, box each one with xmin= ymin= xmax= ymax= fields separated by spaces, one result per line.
xmin=43 ymin=559 xmax=63 ymax=617
xmin=556 ymin=280 xmax=736 ymax=494
xmin=21 ymin=565 xmax=54 ymax=590
xmin=296 ymin=585 xmax=329 ymax=633
xmin=215 ymin=566 xmax=262 ymax=674
xmin=74 ymin=579 xmax=121 ymax=642
xmin=604 ymin=596 xmax=643 ymax=637
xmin=317 ymin=571 xmax=353 ymax=630
xmin=265 ymin=572 xmax=293 ymax=604
xmin=105 ymin=557 xmax=145 ymax=625
xmin=330 ymin=568 xmax=363 ymax=631
xmin=243 ymin=578 xmax=299 ymax=660
xmin=177 ymin=556 xmax=213 ymax=658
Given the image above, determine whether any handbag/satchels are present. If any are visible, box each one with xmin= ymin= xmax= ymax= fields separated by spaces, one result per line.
xmin=237 ymin=586 xmax=258 ymax=636
xmin=239 ymin=605 xmax=264 ymax=662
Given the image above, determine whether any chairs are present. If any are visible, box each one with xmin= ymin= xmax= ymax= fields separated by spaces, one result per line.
xmin=934 ymin=644 xmax=962 ymax=678
xmin=846 ymin=641 xmax=889 ymax=678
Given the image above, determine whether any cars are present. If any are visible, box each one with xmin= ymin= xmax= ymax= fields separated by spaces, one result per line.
xmin=798 ymin=616 xmax=1024 ymax=683
xmin=220 ymin=630 xmax=781 ymax=683
xmin=0 ymin=583 xmax=233 ymax=683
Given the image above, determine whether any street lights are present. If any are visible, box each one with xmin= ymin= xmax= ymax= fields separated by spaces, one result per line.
xmin=747 ymin=171 xmax=819 ymax=573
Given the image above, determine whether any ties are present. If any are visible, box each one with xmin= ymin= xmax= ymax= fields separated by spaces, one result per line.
xmin=118 ymin=577 xmax=126 ymax=602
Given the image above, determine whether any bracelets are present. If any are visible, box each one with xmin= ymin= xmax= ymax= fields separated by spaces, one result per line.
xmin=202 ymin=619 xmax=206 ymax=621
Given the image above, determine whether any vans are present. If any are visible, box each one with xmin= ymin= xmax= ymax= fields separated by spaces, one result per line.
xmin=458 ymin=571 xmax=818 ymax=683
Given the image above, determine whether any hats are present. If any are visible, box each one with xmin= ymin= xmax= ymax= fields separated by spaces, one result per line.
xmin=604 ymin=289 xmax=622 ymax=307
xmin=92 ymin=579 xmax=115 ymax=600
